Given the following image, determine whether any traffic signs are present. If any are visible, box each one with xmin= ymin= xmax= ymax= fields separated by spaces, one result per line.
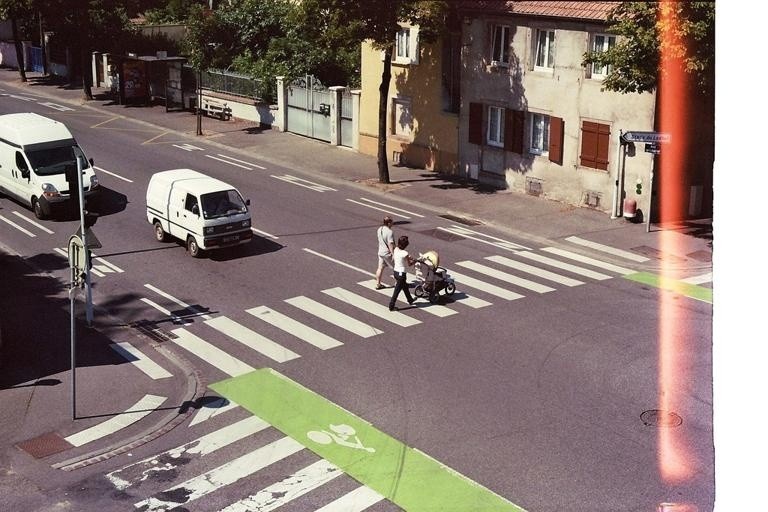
xmin=621 ymin=130 xmax=670 ymax=144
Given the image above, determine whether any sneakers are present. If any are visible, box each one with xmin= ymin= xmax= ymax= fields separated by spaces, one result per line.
xmin=410 ymin=301 xmax=417 ymax=305
xmin=376 ymin=285 xmax=385 ymax=289
xmin=389 ymin=307 xmax=398 ymax=311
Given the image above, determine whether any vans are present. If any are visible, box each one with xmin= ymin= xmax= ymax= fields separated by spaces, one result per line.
xmin=0 ymin=111 xmax=101 ymax=220
xmin=146 ymin=168 xmax=253 ymax=258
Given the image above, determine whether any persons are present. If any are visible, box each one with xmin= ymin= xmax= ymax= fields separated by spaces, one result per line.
xmin=375 ymin=216 xmax=397 ymax=290
xmin=388 ymin=235 xmax=418 ymax=311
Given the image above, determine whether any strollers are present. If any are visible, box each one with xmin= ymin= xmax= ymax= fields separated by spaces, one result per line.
xmin=408 ymin=250 xmax=455 ymax=304
